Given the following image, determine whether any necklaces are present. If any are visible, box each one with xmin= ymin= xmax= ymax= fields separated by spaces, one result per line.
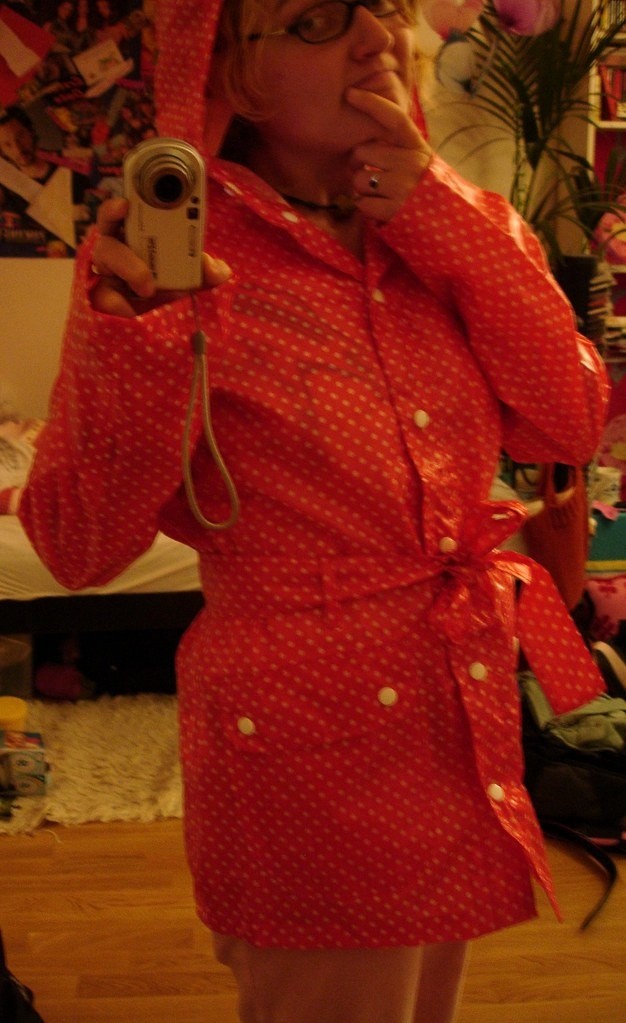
xmin=275 ymin=189 xmax=358 ymax=223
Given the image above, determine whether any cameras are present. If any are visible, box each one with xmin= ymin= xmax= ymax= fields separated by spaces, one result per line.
xmin=123 ymin=136 xmax=205 ymax=290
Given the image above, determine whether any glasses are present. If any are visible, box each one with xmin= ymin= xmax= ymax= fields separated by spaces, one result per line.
xmin=213 ymin=0 xmax=401 ymax=52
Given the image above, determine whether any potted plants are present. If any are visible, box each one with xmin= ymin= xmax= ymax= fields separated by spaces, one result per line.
xmin=421 ymin=0 xmax=626 ymax=325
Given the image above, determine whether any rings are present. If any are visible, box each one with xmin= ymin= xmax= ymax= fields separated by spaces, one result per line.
xmin=368 ymin=174 xmax=382 ymax=187
xmin=91 ymin=264 xmax=102 ymax=275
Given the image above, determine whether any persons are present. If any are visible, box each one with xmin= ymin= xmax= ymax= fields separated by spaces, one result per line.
xmin=21 ymin=0 xmax=606 ymax=1023
